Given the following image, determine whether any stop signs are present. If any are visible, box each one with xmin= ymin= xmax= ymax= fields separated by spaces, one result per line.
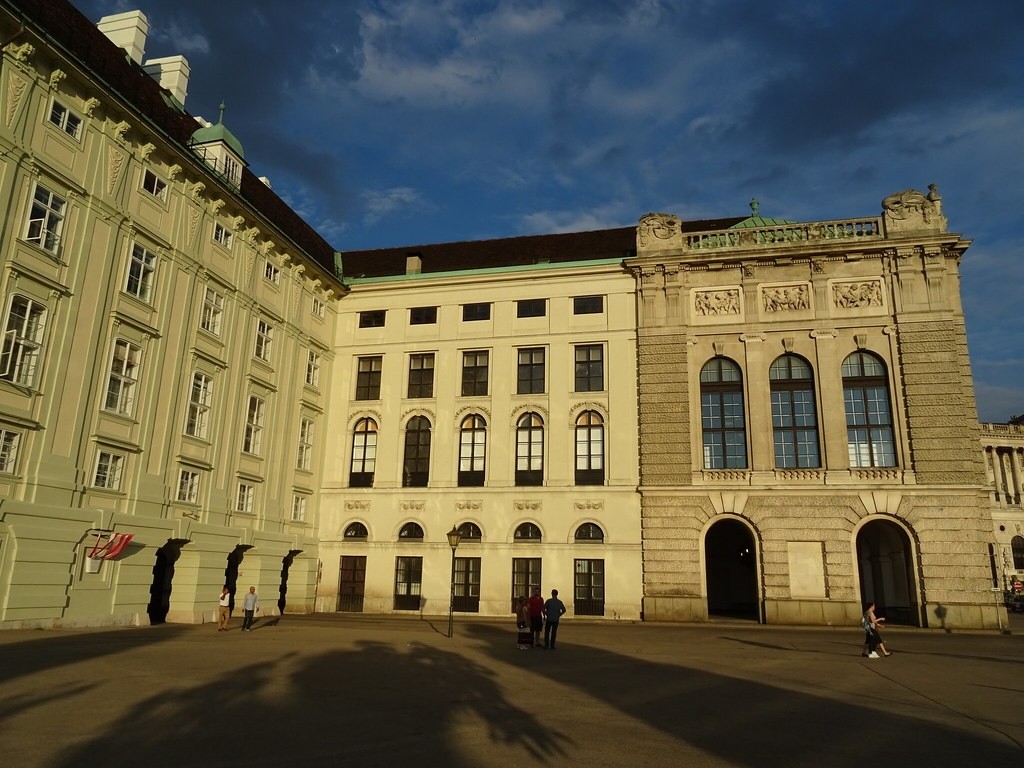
xmin=1014 ymin=583 xmax=1022 ymax=590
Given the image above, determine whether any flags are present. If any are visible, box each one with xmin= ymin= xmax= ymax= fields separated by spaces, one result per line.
xmin=104 ymin=534 xmax=126 ymax=558
xmin=88 ymin=532 xmax=102 ymax=558
xmin=110 ymin=534 xmax=134 ymax=560
xmin=95 ymin=534 xmax=118 ymax=557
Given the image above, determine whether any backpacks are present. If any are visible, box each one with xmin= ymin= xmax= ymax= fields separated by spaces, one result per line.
xmin=859 ymin=616 xmax=873 ymax=636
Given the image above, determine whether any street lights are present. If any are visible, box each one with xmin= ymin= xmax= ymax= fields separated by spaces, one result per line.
xmin=446 ymin=525 xmax=462 ymax=638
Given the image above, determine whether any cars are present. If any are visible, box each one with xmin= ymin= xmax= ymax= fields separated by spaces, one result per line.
xmin=1003 ymin=592 xmax=1024 ymax=612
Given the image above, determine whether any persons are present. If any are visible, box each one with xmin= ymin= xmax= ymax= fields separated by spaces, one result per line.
xmin=860 ymin=602 xmax=893 ymax=660
xmin=217 ymin=585 xmax=232 ymax=631
xmin=242 ymin=586 xmax=260 ymax=631
xmin=515 ymin=587 xmax=565 ymax=650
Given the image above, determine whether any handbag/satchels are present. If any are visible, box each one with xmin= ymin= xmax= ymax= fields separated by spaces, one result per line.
xmin=518 ymin=627 xmax=534 ymax=644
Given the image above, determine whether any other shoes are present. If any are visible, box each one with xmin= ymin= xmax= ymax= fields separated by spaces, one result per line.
xmin=550 ymin=645 xmax=556 ymax=650
xmin=868 ymin=651 xmax=880 ymax=659
xmin=246 ymin=628 xmax=250 ymax=631
xmin=862 ymin=652 xmax=869 ymax=657
xmin=544 ymin=644 xmax=549 ymax=648
xmin=218 ymin=628 xmax=222 ymax=631
xmin=884 ymin=652 xmax=892 ymax=657
xmin=241 ymin=628 xmax=244 ymax=631
xmin=517 ymin=644 xmax=528 ymax=650
xmin=535 ymin=642 xmax=541 ymax=648
xmin=222 ymin=628 xmax=227 ymax=631
